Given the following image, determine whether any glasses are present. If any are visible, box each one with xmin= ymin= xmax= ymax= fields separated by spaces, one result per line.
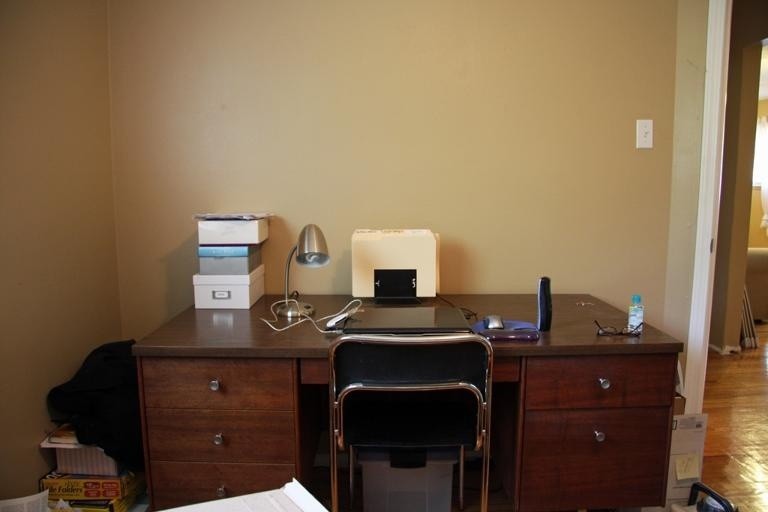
xmin=593 ymin=320 xmax=643 ymax=337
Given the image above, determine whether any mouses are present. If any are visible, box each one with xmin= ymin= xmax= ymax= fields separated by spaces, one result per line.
xmin=483 ymin=313 xmax=504 ymax=330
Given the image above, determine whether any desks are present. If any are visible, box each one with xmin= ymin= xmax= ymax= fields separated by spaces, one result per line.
xmin=131 ymin=293 xmax=686 ymax=512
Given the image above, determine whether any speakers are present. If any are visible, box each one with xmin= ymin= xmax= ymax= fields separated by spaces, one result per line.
xmin=537 ymin=275 xmax=553 ymax=331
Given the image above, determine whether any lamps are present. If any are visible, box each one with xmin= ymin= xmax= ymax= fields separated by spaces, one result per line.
xmin=276 ymin=224 xmax=330 ymax=318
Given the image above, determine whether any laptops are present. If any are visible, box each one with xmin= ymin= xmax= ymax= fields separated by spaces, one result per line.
xmin=342 ymin=306 xmax=473 ymax=335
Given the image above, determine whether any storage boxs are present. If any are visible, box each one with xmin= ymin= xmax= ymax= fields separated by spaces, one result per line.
xmin=190 ymin=211 xmax=280 ymax=310
xmin=38 ymin=423 xmax=146 ymax=511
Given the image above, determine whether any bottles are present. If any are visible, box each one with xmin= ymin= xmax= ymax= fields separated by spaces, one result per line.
xmin=628 ymin=295 xmax=644 ymax=335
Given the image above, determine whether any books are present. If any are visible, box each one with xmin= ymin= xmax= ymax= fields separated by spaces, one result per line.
xmin=39 ymin=423 xmax=80 ymax=449
